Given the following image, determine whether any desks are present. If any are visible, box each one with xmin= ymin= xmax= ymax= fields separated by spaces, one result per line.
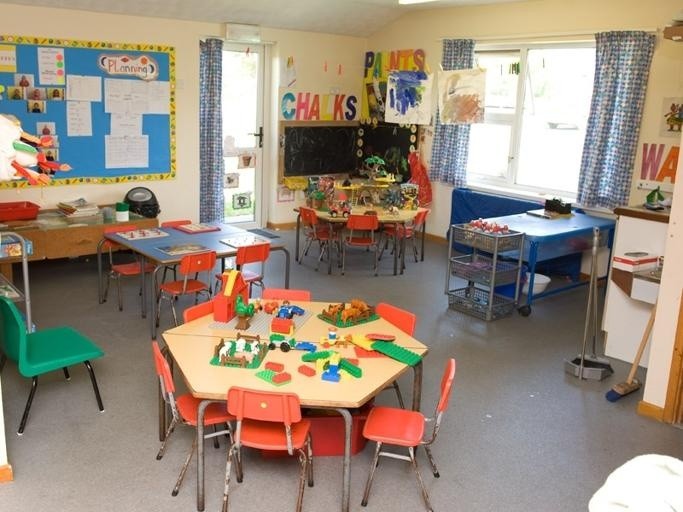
xmin=0 ymin=202 xmax=159 ymax=286
xmin=294 ymin=207 xmax=417 ymax=275
xmin=158 ymin=298 xmax=428 ymax=456
xmin=97 ymin=219 xmax=291 ymax=340
xmin=470 ymin=207 xmax=615 ymax=318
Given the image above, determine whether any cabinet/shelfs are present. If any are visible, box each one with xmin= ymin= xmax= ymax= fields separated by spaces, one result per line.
xmin=0 ymin=225 xmax=35 ymax=333
xmin=600 ymin=204 xmax=670 ymax=372
xmin=445 ymin=221 xmax=525 ymax=321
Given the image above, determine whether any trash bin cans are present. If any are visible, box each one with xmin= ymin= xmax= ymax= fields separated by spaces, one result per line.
xmin=124 ymin=187 xmax=159 ymax=218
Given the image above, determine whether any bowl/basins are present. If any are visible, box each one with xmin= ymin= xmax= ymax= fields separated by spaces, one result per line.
xmin=522 ymin=271 xmax=551 ymax=295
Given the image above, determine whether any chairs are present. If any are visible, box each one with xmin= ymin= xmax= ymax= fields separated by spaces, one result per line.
xmin=377 ymin=301 xmax=417 ymax=338
xmin=182 ymin=301 xmax=212 ymax=322
xmin=359 ymin=357 xmax=457 ymax=512
xmin=297 ymin=207 xmax=341 ymax=273
xmin=341 ymin=213 xmax=380 ymax=278
xmin=262 ymin=288 xmax=311 ymax=303
xmin=0 ymin=295 xmax=106 ymax=437
xmin=102 ymin=225 xmax=155 ymax=310
xmin=155 ymin=251 xmax=217 ymax=328
xmin=214 ymin=243 xmax=270 ymax=302
xmin=151 ymin=338 xmax=243 ymax=496
xmin=379 ymin=210 xmax=428 ymax=269
xmin=220 ymin=386 xmax=314 ymax=512
xmin=160 ymin=219 xmax=199 ymax=299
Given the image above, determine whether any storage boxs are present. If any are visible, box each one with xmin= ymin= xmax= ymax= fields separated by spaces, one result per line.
xmin=260 ymin=396 xmax=376 ymax=461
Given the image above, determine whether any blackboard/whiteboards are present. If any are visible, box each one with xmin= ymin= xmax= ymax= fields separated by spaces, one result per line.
xmin=279 ymin=121 xmax=420 ymax=183
xmin=0 ymin=36 xmax=176 ymax=188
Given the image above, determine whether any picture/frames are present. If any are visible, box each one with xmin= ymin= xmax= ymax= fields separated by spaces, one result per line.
xmin=658 ymin=96 xmax=683 ymax=139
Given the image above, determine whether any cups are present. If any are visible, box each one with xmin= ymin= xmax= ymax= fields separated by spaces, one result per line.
xmin=103 ymin=207 xmax=113 ymax=223
xmin=655 ymin=255 xmax=663 ymax=278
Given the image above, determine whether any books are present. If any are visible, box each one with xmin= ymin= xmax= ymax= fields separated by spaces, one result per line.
xmin=56 ymin=198 xmax=100 ymax=219
